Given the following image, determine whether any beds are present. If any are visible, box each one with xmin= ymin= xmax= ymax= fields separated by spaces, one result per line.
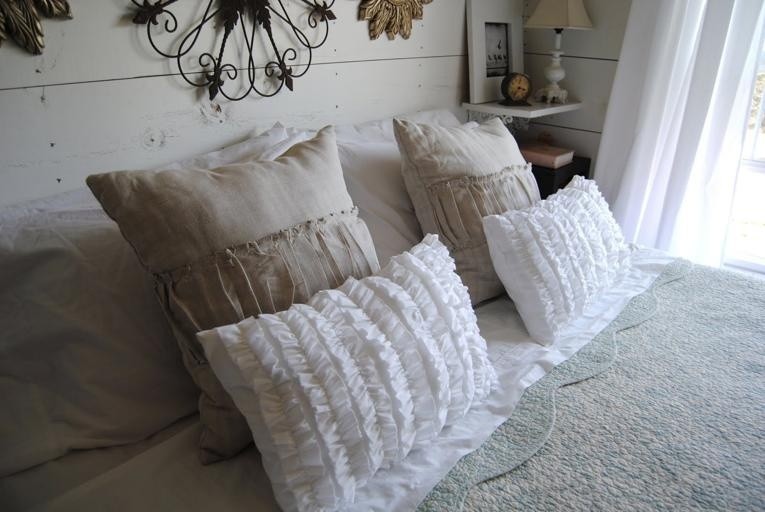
xmin=0 ymin=243 xmax=765 ymax=512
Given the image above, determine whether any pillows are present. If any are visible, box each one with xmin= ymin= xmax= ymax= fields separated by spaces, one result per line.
xmin=394 ymin=117 xmax=542 ymax=309
xmin=334 ymin=140 xmax=424 ymax=270
xmin=194 ymin=234 xmax=498 ymax=512
xmin=481 ymin=175 xmax=631 ymax=344
xmin=0 ymin=208 xmax=199 ymax=481
xmin=85 ymin=125 xmax=383 ymax=466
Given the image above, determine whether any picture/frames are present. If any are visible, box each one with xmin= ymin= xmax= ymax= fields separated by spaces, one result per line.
xmin=465 ymin=0 xmax=528 ymax=104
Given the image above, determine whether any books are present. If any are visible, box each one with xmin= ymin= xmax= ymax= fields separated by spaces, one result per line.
xmin=520 ymin=142 xmax=576 ymax=169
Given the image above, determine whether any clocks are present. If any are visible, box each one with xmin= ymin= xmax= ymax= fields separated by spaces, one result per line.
xmin=498 ymin=72 xmax=534 ymax=105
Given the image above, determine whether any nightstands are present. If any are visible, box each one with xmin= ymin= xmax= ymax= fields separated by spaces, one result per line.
xmin=522 ymin=156 xmax=592 ymax=199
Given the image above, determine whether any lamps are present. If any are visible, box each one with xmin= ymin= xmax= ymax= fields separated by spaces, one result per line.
xmin=521 ymin=0 xmax=594 ymax=104
